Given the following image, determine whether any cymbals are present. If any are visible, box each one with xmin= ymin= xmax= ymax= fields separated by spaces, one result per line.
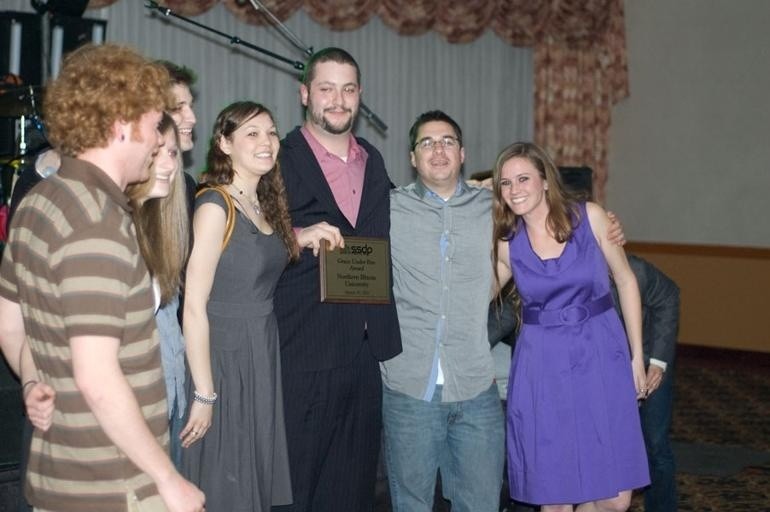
xmin=0 ymin=83 xmax=44 ymax=116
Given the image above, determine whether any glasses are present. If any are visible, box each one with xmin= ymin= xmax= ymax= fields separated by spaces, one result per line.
xmin=413 ymin=138 xmax=457 ymax=151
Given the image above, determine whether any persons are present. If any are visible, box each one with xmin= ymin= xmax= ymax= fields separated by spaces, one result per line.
xmin=373 ymin=104 xmax=630 ymax=511
xmin=467 ymin=166 xmax=683 ymax=510
xmin=177 ymin=99 xmax=309 ymax=511
xmin=16 ymin=111 xmax=190 ymax=479
xmin=0 ymin=141 xmax=61 ymax=512
xmin=267 ymin=44 xmax=406 ymax=510
xmin=147 ymin=58 xmax=202 ymax=213
xmin=487 ymin=142 xmax=649 ymax=512
xmin=0 ymin=42 xmax=211 ymax=512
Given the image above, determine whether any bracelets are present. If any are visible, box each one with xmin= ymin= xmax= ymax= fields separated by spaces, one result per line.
xmin=190 ymin=390 xmax=219 ymax=408
xmin=19 ymin=379 xmax=36 ymax=391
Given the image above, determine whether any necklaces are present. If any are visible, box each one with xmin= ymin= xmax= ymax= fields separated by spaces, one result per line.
xmin=233 ymin=183 xmax=263 ymax=218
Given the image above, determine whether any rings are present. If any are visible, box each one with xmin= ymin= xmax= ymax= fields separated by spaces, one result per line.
xmin=639 ymin=386 xmax=647 ymax=393
xmin=191 ymin=429 xmax=200 ymax=436
xmin=651 ymin=382 xmax=659 ymax=387
